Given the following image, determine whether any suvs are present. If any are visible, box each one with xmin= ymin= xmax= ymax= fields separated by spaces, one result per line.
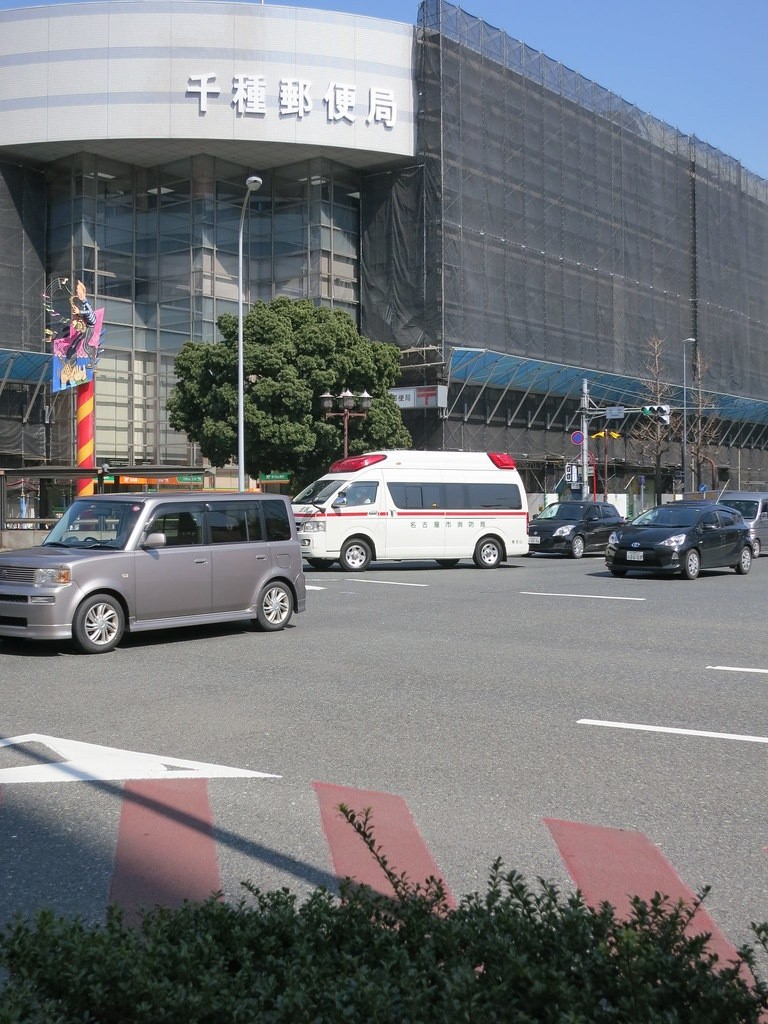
xmin=0 ymin=488 xmax=308 ymax=655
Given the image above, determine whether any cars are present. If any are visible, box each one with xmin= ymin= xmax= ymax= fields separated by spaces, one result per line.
xmin=521 ymin=500 xmax=627 ymax=559
xmin=603 ymin=502 xmax=754 ymax=581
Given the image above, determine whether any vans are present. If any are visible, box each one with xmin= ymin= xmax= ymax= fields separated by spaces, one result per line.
xmin=717 ymin=490 xmax=768 ymax=559
xmin=288 ymin=451 xmax=531 ymax=573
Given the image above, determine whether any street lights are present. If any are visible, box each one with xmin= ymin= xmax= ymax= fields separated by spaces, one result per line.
xmin=591 ymin=429 xmax=624 ymax=502
xmin=318 ymin=386 xmax=374 ymax=458
xmin=682 ymin=337 xmax=695 ymax=495
xmin=234 ymin=175 xmax=268 ymax=492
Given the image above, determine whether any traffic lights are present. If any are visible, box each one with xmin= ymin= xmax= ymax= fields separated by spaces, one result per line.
xmin=653 ymin=415 xmax=670 ymax=426
xmin=641 ymin=404 xmax=669 ymax=416
xmin=564 ymin=464 xmax=571 ymax=482
